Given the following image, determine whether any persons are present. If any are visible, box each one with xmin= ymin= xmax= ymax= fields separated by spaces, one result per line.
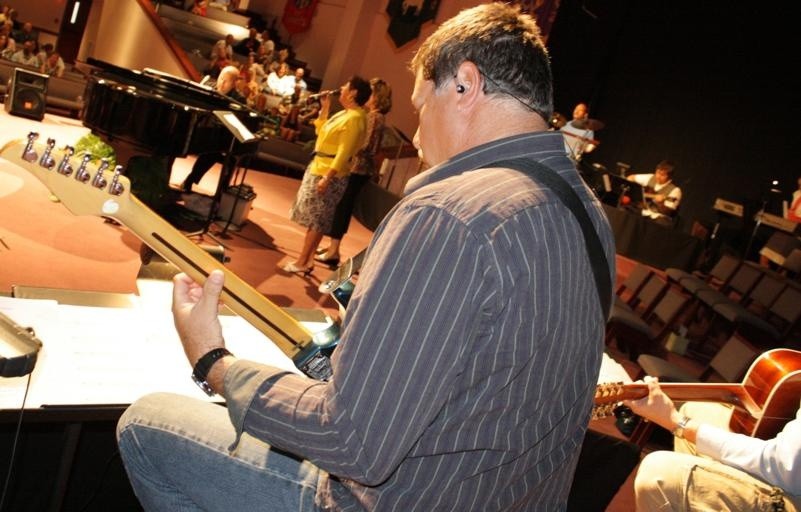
xmin=627 ymin=159 xmax=683 ymax=230
xmin=1 ymin=5 xmax=66 ymax=78
xmin=117 ymin=1 xmax=618 ymax=511
xmin=182 ymin=66 xmax=246 ymax=194
xmin=277 ymin=74 xmax=371 ymax=278
xmin=760 ymin=176 xmax=801 ymax=269
xmin=563 ymin=103 xmax=600 ymax=163
xmin=314 ymin=76 xmax=393 ymax=269
xmin=210 ymin=27 xmax=321 ymax=143
xmin=603 ymin=373 xmax=801 ymax=511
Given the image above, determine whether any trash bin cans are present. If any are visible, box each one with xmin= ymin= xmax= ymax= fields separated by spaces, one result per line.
xmin=220 ymin=184 xmax=256 ymax=224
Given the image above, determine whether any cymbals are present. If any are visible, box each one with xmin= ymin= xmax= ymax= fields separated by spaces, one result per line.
xmin=571 ymin=118 xmax=605 ymax=131
xmin=547 ymin=112 xmax=567 ymax=129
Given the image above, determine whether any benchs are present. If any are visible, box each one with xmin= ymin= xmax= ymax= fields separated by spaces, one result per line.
xmin=156 ymin=0 xmax=256 ymax=73
xmin=1 ymin=59 xmax=84 ymax=120
xmin=258 ymin=131 xmax=309 ymax=173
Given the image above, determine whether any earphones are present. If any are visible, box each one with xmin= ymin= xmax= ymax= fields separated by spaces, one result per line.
xmin=457 ymin=84 xmax=465 ymax=93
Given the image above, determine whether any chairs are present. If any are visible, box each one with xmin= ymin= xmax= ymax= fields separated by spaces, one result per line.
xmin=607 ymin=247 xmax=799 ymax=383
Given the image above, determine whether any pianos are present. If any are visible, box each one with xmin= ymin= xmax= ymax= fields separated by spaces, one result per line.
xmin=712 ymin=198 xmax=800 ymax=237
xmin=83 ymin=55 xmax=260 ymax=234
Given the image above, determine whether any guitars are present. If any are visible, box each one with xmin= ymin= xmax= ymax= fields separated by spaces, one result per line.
xmin=624 ymin=185 xmax=677 ymax=208
xmin=590 ymin=349 xmax=801 ymax=439
xmin=1 ymin=133 xmax=356 ymax=383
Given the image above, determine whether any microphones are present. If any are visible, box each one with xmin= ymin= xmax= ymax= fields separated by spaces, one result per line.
xmin=319 ymin=90 xmax=339 ymax=105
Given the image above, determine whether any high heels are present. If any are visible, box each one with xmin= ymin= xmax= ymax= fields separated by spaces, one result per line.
xmin=314 ymin=248 xmax=340 ymax=268
xmin=279 ymin=261 xmax=314 ymax=278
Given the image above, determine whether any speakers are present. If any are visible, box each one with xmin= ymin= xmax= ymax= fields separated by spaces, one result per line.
xmin=4 ymin=67 xmax=50 ymax=121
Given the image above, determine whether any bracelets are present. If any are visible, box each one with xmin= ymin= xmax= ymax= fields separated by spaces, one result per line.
xmin=192 ymin=347 xmax=236 ymax=397
xmin=323 ymin=176 xmax=329 ymax=181
xmin=673 ymin=415 xmax=691 ymax=439
